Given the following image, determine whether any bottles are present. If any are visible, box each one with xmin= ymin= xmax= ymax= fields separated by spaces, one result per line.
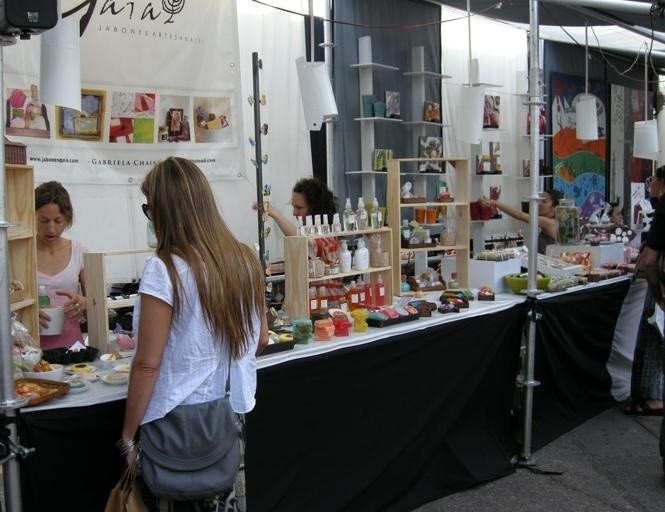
xmin=342 ymin=195 xmax=383 ymax=232
xmin=309 ymin=273 xmax=385 ymax=318
xmin=38 ymin=285 xmax=50 ymax=308
xmin=449 ymin=272 xmax=459 ymax=288
xmin=400 ymin=274 xmax=411 ymax=292
xmin=555 ymin=194 xmax=582 ymax=246
xmin=440 ymin=215 xmax=456 ymax=246
xmin=297 ymin=213 xmax=342 ymax=237
xmin=401 ymin=219 xmax=411 ymax=247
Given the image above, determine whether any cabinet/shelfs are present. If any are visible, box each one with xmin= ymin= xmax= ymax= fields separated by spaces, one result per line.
xmin=84 ymin=249 xmax=154 ymax=362
xmin=387 ymin=158 xmax=472 ymax=294
xmin=345 ymin=36 xmax=454 ymax=228
xmin=283 ymin=228 xmax=394 ymax=321
xmin=466 ymin=59 xmax=555 ymax=222
xmin=4 ymin=165 xmax=41 ymax=363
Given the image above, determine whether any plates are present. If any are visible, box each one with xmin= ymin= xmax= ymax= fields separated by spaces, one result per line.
xmin=64 ymin=364 xmax=99 ymax=374
xmin=63 ymin=379 xmax=89 ymax=393
xmin=113 ymin=363 xmax=129 ymax=372
xmin=101 ymin=372 xmax=128 ymax=386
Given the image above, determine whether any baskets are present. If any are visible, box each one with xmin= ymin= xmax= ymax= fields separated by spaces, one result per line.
xmin=13 ymin=378 xmax=71 ymax=407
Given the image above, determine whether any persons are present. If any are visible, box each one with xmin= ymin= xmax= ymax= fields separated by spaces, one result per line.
xmin=479 ymin=188 xmax=565 ymax=256
xmin=75 ymin=302 xmax=81 ymax=308
xmin=619 ymin=163 xmax=665 ymax=417
xmin=118 ymin=156 xmax=273 ymax=512
xmin=34 ymin=178 xmax=93 ymax=354
xmin=606 ymin=203 xmax=626 ymax=229
xmin=250 ymin=176 xmax=341 ymax=239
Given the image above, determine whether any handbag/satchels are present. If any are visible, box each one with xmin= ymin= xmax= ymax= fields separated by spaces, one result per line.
xmin=106 ymin=463 xmax=148 ymax=511
xmin=140 ymin=398 xmax=240 ymax=498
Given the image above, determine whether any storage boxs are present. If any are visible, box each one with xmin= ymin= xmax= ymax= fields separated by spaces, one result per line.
xmin=545 ymin=242 xmax=625 ymax=272
xmin=469 ymin=257 xmax=522 ymax=294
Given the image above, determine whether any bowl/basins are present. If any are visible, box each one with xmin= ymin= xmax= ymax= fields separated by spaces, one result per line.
xmin=23 ymin=363 xmax=63 ymax=382
xmin=505 ymin=272 xmax=551 ymax=296
xmin=100 ymin=353 xmax=116 ymax=370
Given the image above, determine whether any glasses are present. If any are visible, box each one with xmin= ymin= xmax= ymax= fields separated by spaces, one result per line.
xmin=140 ymin=205 xmax=152 ymax=218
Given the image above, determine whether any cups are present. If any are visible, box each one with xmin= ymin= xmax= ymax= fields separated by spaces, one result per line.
xmin=39 ymin=305 xmax=66 ymax=337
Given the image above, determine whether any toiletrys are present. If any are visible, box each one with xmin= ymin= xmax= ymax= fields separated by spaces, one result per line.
xmin=355 ymin=197 xmax=368 ymax=231
xmin=339 ymin=239 xmax=351 ymax=273
xmin=377 ymin=275 xmax=386 ymax=306
xmin=316 ymin=318 xmax=335 ymax=342
xmin=342 ymin=197 xmax=356 ymax=232
xmin=437 ymin=175 xmax=448 ymax=199
xmin=355 ymin=238 xmax=370 ymax=270
xmin=440 ymin=221 xmax=456 ymax=246
xmin=349 ymin=278 xmax=371 ymax=311
xmin=371 ymin=197 xmax=382 ymax=229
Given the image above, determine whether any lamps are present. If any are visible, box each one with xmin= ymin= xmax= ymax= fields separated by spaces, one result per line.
xmin=576 ymin=18 xmax=598 ymax=142
xmin=295 ymin=0 xmax=338 ymax=133
xmin=37 ymin=0 xmax=83 ymax=113
xmin=632 ymin=45 xmax=660 ymax=163
xmin=457 ymin=2 xmax=484 ymax=144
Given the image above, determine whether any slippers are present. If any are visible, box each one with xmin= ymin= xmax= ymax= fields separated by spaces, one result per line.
xmin=624 ymin=401 xmax=665 ymax=415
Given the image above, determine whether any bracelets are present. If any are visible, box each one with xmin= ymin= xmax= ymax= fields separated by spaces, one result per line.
xmin=115 ymin=437 xmax=137 ymax=457
